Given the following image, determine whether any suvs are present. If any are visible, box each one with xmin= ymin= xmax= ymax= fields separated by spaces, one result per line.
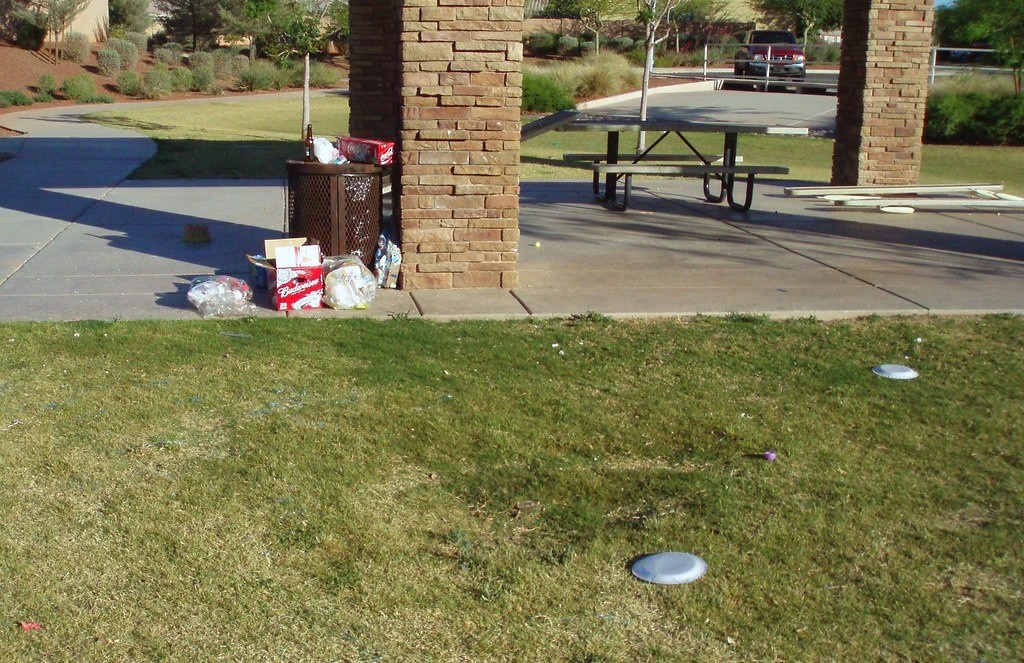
xmin=734 ymin=30 xmax=806 ymax=79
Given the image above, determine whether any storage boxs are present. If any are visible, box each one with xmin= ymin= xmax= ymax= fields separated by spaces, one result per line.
xmin=335 ymin=136 xmax=395 ymax=166
xmin=246 ymin=237 xmax=324 ymax=311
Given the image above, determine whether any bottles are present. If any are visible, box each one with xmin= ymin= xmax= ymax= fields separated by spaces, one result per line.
xmin=304 ymin=124 xmax=314 ymax=161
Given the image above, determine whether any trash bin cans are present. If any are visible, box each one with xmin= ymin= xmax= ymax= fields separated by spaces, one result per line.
xmin=285 ymin=159 xmax=385 ymax=276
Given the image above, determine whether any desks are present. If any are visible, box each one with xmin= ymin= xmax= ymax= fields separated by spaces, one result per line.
xmin=552 ymin=120 xmax=810 ymax=211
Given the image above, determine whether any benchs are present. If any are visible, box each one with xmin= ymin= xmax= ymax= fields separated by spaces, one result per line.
xmin=562 ymin=154 xmax=744 ymax=162
xmin=590 ymin=163 xmax=790 ymax=174
